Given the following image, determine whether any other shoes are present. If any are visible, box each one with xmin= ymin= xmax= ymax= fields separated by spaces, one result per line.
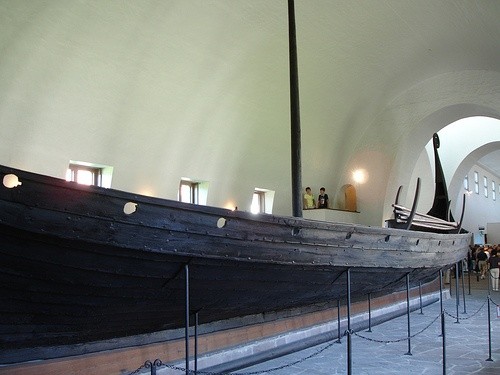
xmin=464 ymin=269 xmax=487 ymax=280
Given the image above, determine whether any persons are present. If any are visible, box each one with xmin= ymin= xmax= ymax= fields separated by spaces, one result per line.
xmin=468 ymin=242 xmax=500 ymax=291
xmin=303 ymin=187 xmax=316 ymax=210
xmin=318 ymin=188 xmax=328 ymax=208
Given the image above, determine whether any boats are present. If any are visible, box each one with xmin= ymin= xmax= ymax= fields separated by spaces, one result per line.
xmin=0 ymin=0 xmax=474 ymax=350
